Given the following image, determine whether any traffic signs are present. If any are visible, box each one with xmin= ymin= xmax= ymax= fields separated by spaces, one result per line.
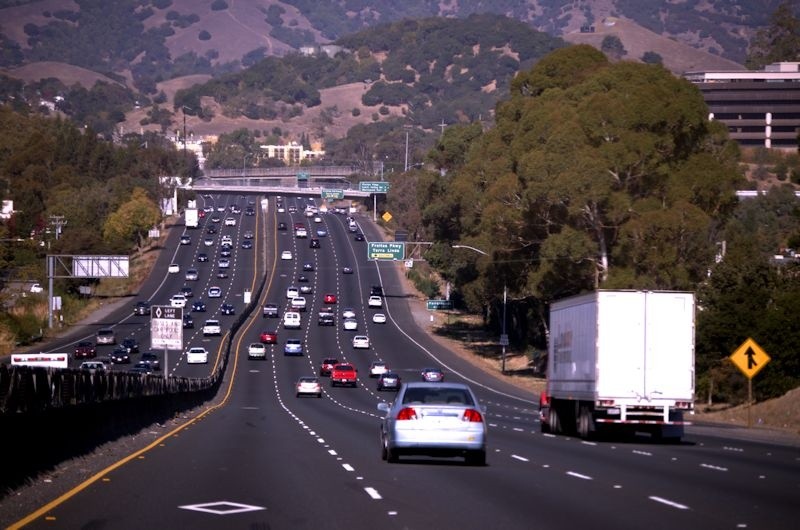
xmin=367 ymin=241 xmax=407 ymax=262
xmin=359 ymin=180 xmax=390 ymax=194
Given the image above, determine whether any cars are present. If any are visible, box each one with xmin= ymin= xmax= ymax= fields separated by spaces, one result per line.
xmin=126 ymin=366 xmax=152 ymax=375
xmin=293 ymin=376 xmax=323 ymax=399
xmin=130 ymin=193 xmax=387 ymax=344
xmin=136 ymin=352 xmax=160 ymax=370
xmin=120 ymin=337 xmax=140 ymax=354
xmin=368 ymin=360 xmax=392 ymax=378
xmin=133 ymin=301 xmax=152 ymax=315
xmin=247 ymin=342 xmax=266 ymax=360
xmin=376 ymin=373 xmax=402 ymax=392
xmin=74 ymin=340 xmax=97 ymax=360
xmin=328 ymin=362 xmax=359 ymax=388
xmin=185 ymin=346 xmax=209 ymax=364
xmin=373 ymin=380 xmax=489 ymax=466
xmin=79 ymin=361 xmax=107 ymax=370
xmin=283 ymin=338 xmax=303 ymax=357
xmin=318 ymin=357 xmax=340 ymax=377
xmin=420 ymin=368 xmax=445 ymax=382
xmin=350 ymin=334 xmax=371 ymax=349
xmin=108 ymin=348 xmax=129 ymax=365
xmin=95 ymin=328 xmax=116 ymax=346
xmin=92 ymin=356 xmax=114 ymax=370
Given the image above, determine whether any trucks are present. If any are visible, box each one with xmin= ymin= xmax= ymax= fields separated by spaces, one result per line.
xmin=539 ymin=289 xmax=698 ymax=447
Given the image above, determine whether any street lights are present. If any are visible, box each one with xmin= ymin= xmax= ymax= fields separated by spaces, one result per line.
xmin=451 ymin=244 xmax=507 ymax=372
